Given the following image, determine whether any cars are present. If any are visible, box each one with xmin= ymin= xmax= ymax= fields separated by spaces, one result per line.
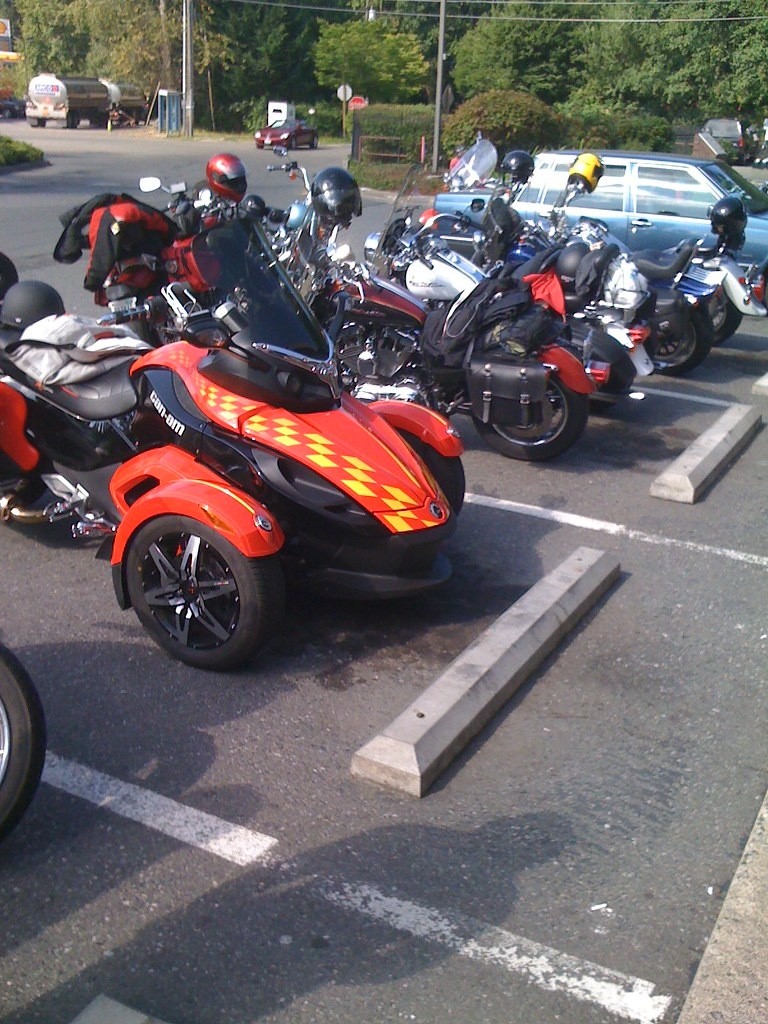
xmin=431 ymin=148 xmax=768 ymax=320
xmin=0 ymin=95 xmax=27 ymax=120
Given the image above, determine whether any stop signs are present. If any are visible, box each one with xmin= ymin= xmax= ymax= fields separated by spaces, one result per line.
xmin=348 ymin=97 xmax=368 ymax=113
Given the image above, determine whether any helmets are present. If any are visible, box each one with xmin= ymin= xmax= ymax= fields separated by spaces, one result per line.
xmin=711 ymin=197 xmax=748 ymax=236
xmin=501 ymin=151 xmax=534 ymax=176
xmin=558 ymin=243 xmax=592 ymax=285
xmin=568 ymin=153 xmax=604 ymax=193
xmin=205 ymin=153 xmax=249 ymax=202
xmin=311 ymin=166 xmax=363 ymax=220
xmin=0 ymin=280 xmax=66 ymax=330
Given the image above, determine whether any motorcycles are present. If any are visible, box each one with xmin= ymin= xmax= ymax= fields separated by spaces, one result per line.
xmin=0 ymin=217 xmax=467 ymax=675
xmin=80 ymin=134 xmax=767 ymax=464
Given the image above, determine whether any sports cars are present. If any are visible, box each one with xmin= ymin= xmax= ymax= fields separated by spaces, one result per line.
xmin=254 ymin=118 xmax=320 ymax=152
